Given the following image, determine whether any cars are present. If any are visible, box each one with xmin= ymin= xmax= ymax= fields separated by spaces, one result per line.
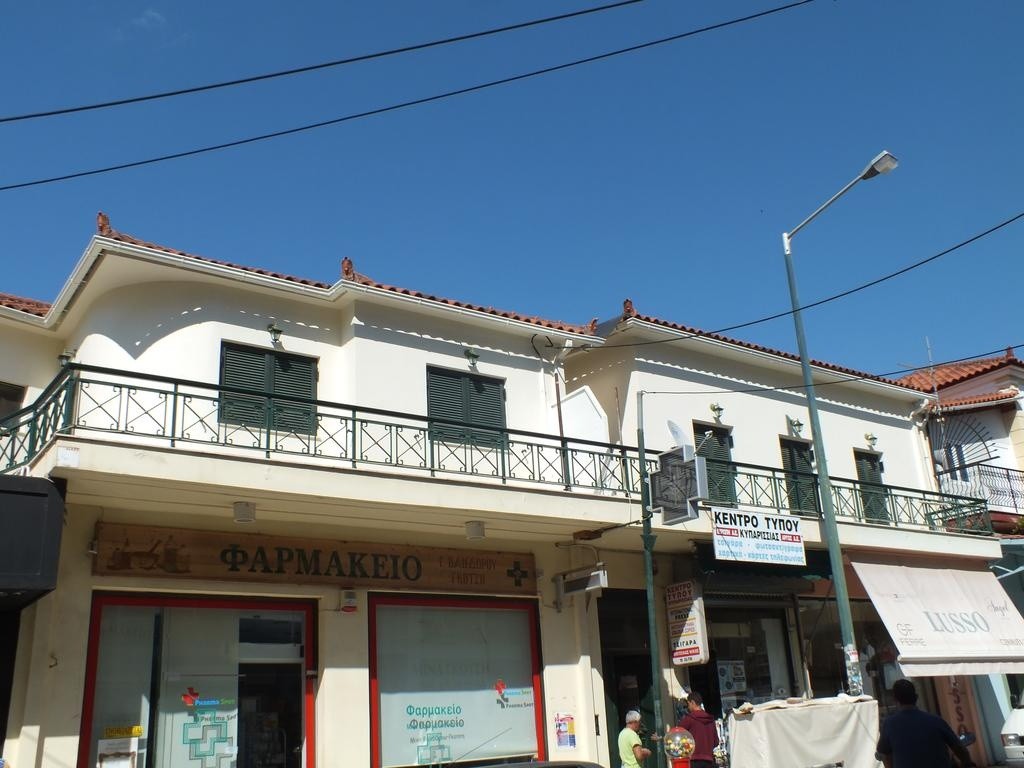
xmin=1000 ymin=689 xmax=1024 ymax=760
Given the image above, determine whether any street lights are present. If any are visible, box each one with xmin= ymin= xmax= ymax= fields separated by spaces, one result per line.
xmin=782 ymin=150 xmax=901 ymax=695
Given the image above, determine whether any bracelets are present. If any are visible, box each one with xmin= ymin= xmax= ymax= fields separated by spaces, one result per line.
xmin=660 ymin=736 xmax=662 ymax=741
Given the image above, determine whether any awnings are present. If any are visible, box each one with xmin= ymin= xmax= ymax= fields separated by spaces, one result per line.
xmin=847 ymin=551 xmax=1024 ymax=677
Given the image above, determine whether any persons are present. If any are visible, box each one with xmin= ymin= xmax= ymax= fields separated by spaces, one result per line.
xmin=651 ymin=693 xmax=719 ymax=768
xmin=875 ymin=679 xmax=973 ymax=768
xmin=618 ymin=711 xmax=651 ymax=768
